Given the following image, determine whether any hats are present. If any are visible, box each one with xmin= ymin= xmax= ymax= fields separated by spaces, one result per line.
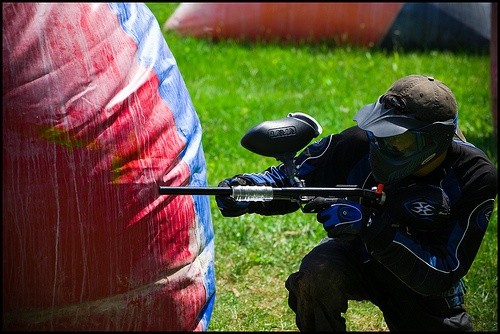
xmin=353 ymin=73 xmax=457 ymax=137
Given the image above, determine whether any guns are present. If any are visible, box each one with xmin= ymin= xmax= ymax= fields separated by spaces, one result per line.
xmin=158 ymin=112 xmax=386 ymax=212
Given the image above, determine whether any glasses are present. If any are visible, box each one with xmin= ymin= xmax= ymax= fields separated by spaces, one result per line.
xmin=380 ymin=128 xmax=424 ymax=158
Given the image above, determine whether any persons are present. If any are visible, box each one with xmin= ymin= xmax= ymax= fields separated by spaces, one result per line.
xmin=216 ymin=74 xmax=499 ymax=334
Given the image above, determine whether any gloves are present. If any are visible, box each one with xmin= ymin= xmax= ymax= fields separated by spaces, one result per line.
xmin=217 ymin=176 xmax=259 ymax=217
xmin=308 ymin=194 xmax=394 ymax=254
